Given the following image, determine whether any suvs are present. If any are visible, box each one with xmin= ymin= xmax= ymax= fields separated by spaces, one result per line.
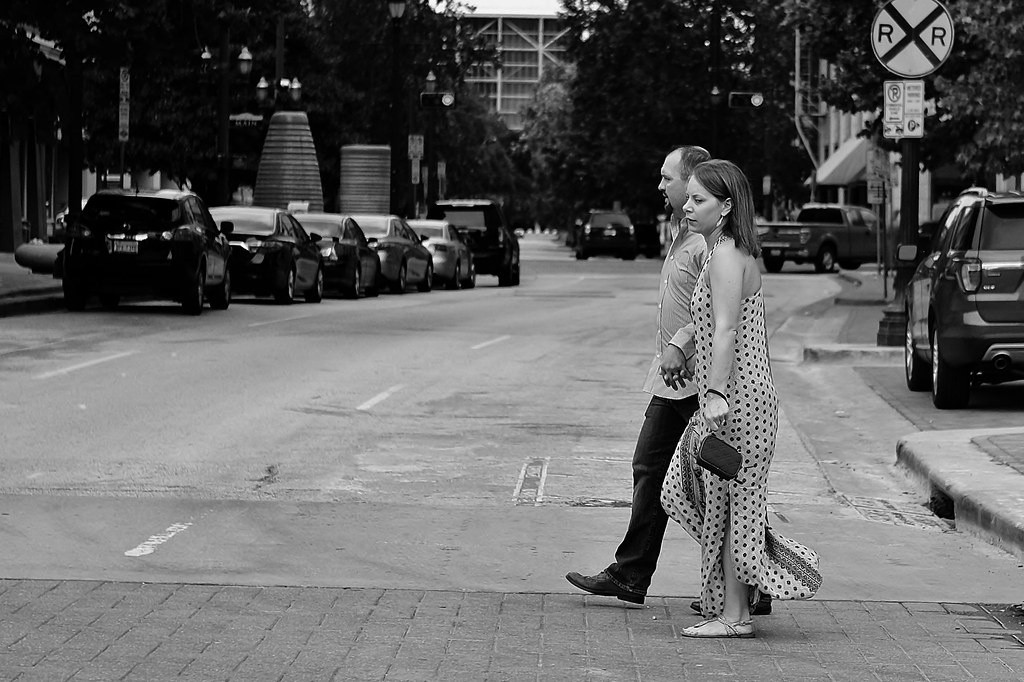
xmin=903 ymin=184 xmax=1024 ymax=409
xmin=573 ymin=207 xmax=637 ymax=260
xmin=426 ymin=196 xmax=525 ymax=286
xmin=60 ymin=187 xmax=235 ymax=315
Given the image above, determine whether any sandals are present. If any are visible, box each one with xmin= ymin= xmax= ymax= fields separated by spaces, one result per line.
xmin=681 ymin=615 xmax=756 ymax=638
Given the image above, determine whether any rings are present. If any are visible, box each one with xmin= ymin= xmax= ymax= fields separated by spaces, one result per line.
xmin=722 ymin=419 xmax=725 ymax=426
xmin=674 ymin=372 xmax=679 ymax=374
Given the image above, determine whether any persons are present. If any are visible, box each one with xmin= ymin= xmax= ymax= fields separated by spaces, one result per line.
xmin=658 ymin=159 xmax=823 ymax=638
xmin=566 ymin=146 xmax=772 ymax=615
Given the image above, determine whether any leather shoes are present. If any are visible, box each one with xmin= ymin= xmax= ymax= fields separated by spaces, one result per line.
xmin=691 ymin=594 xmax=772 ymax=615
xmin=566 ymin=570 xmax=645 ymax=604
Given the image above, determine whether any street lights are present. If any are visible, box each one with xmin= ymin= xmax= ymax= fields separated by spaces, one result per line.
xmin=200 ymin=9 xmax=252 ymax=205
xmin=709 ymin=84 xmax=720 ymax=163
xmin=413 ymin=68 xmax=436 ymax=224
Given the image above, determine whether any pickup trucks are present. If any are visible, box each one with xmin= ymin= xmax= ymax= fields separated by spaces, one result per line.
xmin=753 ymin=201 xmax=884 ymax=274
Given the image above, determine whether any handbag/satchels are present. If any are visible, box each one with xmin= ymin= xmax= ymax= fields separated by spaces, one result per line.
xmin=697 ymin=389 xmax=744 ymax=480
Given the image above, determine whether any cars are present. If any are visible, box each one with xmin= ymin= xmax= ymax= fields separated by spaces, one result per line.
xmin=404 ymin=216 xmax=477 ymax=290
xmin=293 ymin=213 xmax=382 ymax=299
xmin=207 ymin=205 xmax=326 ymax=306
xmin=348 ymin=213 xmax=435 ymax=295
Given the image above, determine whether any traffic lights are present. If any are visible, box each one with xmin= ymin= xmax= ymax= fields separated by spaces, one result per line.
xmin=420 ymin=92 xmax=455 ymax=109
xmin=728 ymin=92 xmax=764 ymax=109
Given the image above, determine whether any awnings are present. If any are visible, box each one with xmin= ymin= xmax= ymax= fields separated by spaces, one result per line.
xmin=804 ymin=137 xmax=866 ymax=185
